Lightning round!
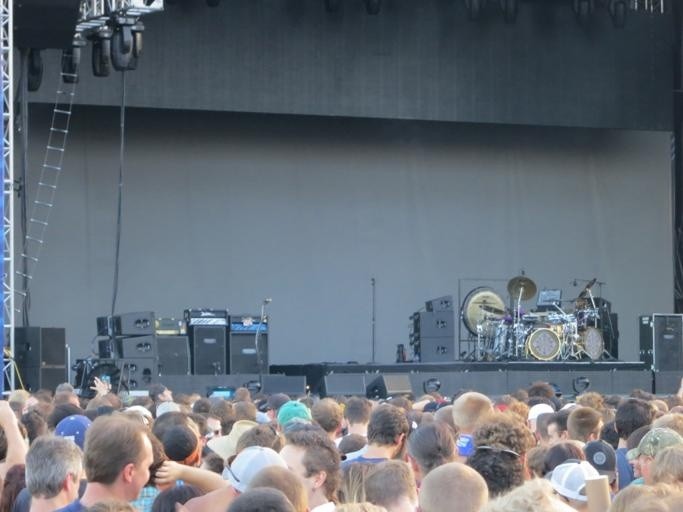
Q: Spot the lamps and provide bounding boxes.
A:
[25,0,143,95]
[362,0,628,31]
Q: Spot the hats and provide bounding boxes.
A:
[206,394,314,492]
[625,428,683,461]
[528,403,553,421]
[550,459,597,501]
[54,414,92,449]
[8,390,38,406]
[585,439,616,484]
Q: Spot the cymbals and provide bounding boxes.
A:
[479,305,504,314]
[507,277,536,300]
[577,278,596,298]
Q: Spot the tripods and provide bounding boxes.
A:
[464,286,532,363]
[551,286,619,361]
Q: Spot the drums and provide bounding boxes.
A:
[525,327,561,360]
[567,322,578,335]
[544,322,563,337]
[578,326,604,360]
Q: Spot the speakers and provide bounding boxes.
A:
[259,374,307,395]
[367,373,413,400]
[310,372,368,400]
[157,335,192,375]
[96,310,160,391]
[227,330,270,376]
[14,326,69,397]
[188,324,228,375]
[413,295,456,363]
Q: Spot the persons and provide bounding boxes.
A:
[1,375,683,510]
[562,297,586,313]
